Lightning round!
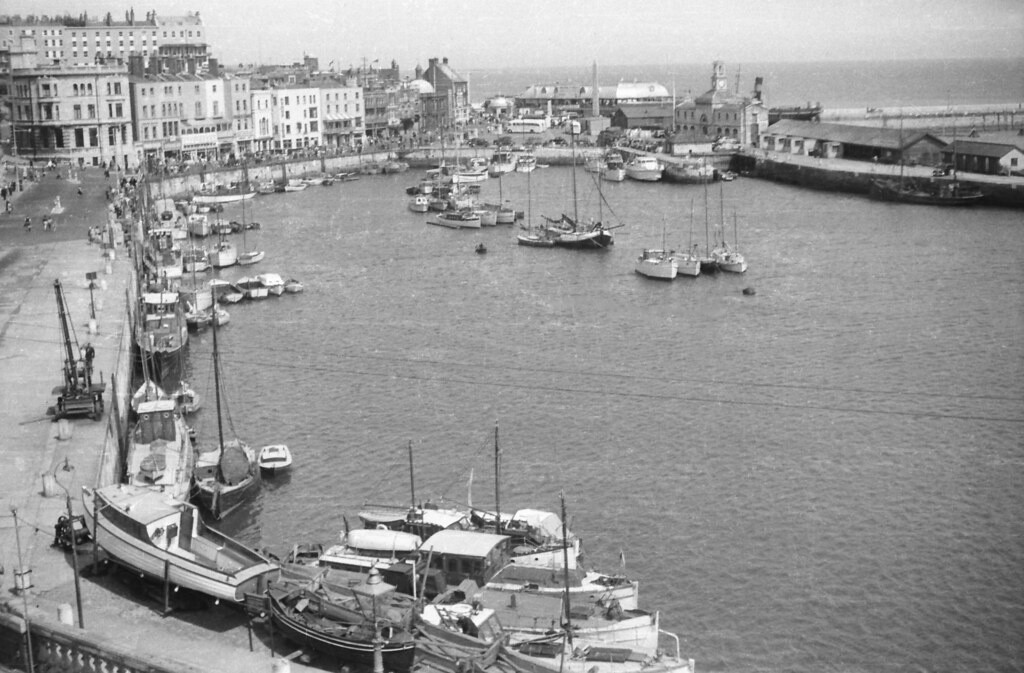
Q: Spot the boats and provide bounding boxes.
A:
[870,102,986,205]
[636,248,680,279]
[673,249,704,278]
[78,483,281,606]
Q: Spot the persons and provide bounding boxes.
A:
[527,116,698,171]
[0,151,141,243]
[84,342,95,376]
[856,149,924,175]
[455,611,479,639]
[146,101,515,181]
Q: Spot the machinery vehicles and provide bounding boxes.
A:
[50,277,107,417]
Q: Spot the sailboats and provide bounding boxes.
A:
[692,178,714,271]
[139,98,735,673]
[709,180,749,273]
